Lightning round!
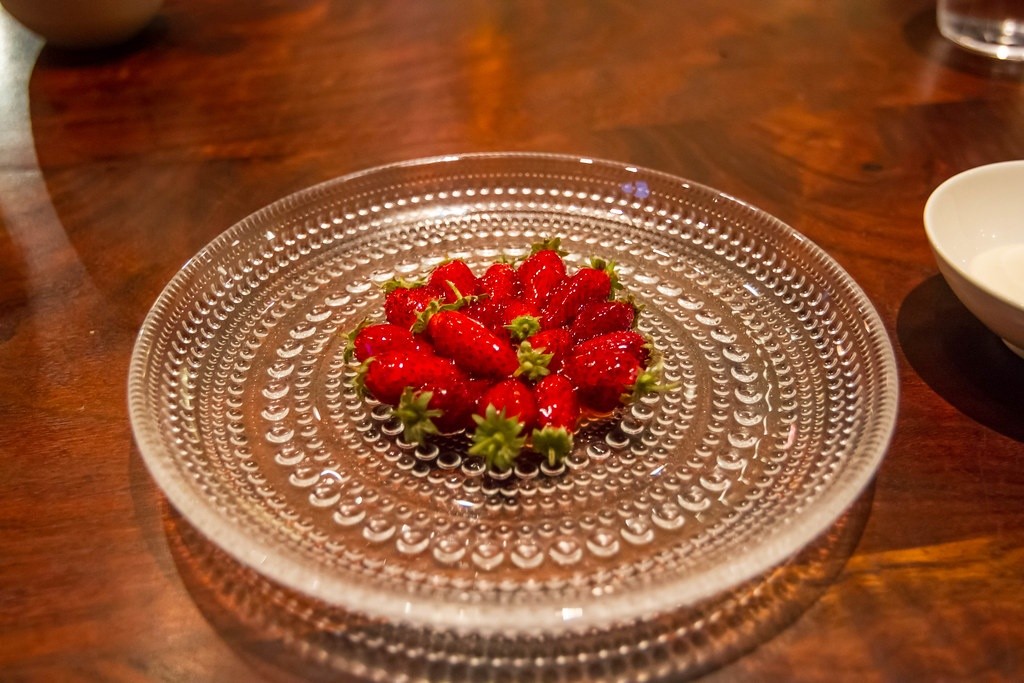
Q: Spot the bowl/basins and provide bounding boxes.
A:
[923,160,1024,359]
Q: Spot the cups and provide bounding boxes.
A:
[937,0,1024,61]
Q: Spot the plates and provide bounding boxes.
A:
[126,151,901,642]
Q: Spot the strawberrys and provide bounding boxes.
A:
[343,236,682,474]
